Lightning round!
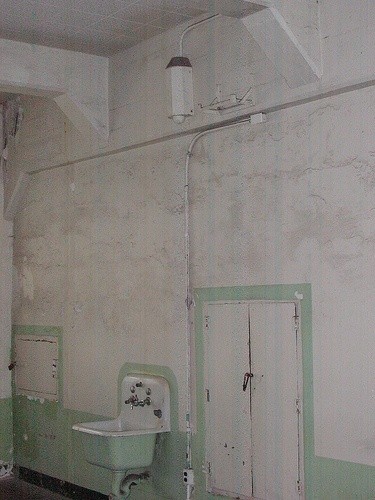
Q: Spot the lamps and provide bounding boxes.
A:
[165,57,194,124]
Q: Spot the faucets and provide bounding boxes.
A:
[129,399,145,410]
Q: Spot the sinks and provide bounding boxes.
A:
[71,418,163,472]
